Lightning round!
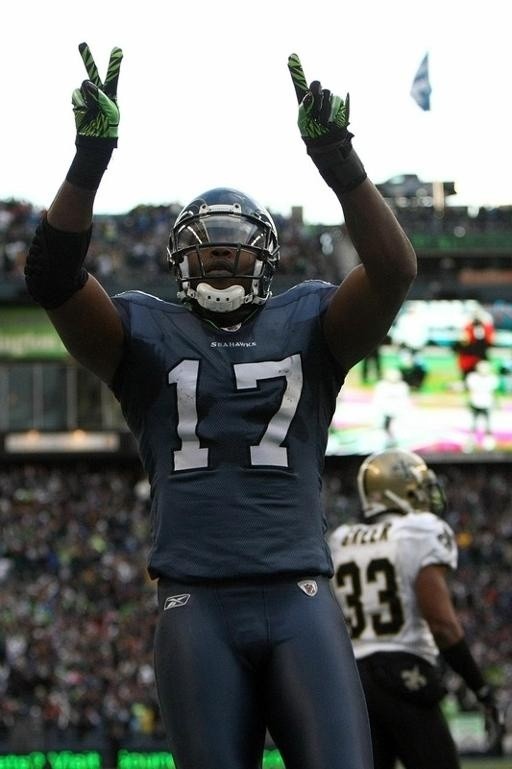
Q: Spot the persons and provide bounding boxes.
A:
[1,197,510,304]
[356,297,501,437]
[26,40,419,769]
[1,446,510,732]
[320,448,507,769]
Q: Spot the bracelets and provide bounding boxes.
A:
[65,146,115,192]
[308,146,367,194]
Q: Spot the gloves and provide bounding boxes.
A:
[289,53,366,193]
[65,42,123,190]
[475,687,503,752]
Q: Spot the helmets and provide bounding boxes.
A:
[165,188,281,333]
[359,450,448,520]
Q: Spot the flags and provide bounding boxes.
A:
[408,57,434,108]
